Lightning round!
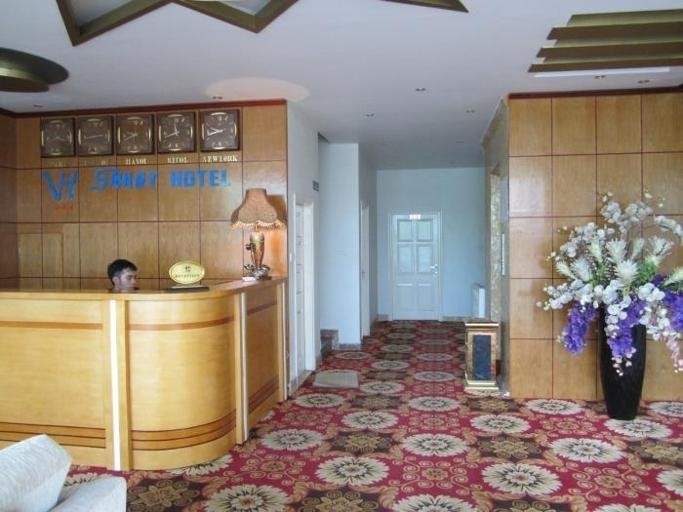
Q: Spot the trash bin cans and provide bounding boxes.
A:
[463,318,500,392]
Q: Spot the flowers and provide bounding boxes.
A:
[532,187,683,377]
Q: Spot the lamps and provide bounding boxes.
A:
[230,186,284,278]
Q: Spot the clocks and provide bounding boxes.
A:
[35,109,242,159]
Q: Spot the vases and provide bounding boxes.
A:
[600,295,646,420]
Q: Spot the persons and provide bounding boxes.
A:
[107,258,138,292]
